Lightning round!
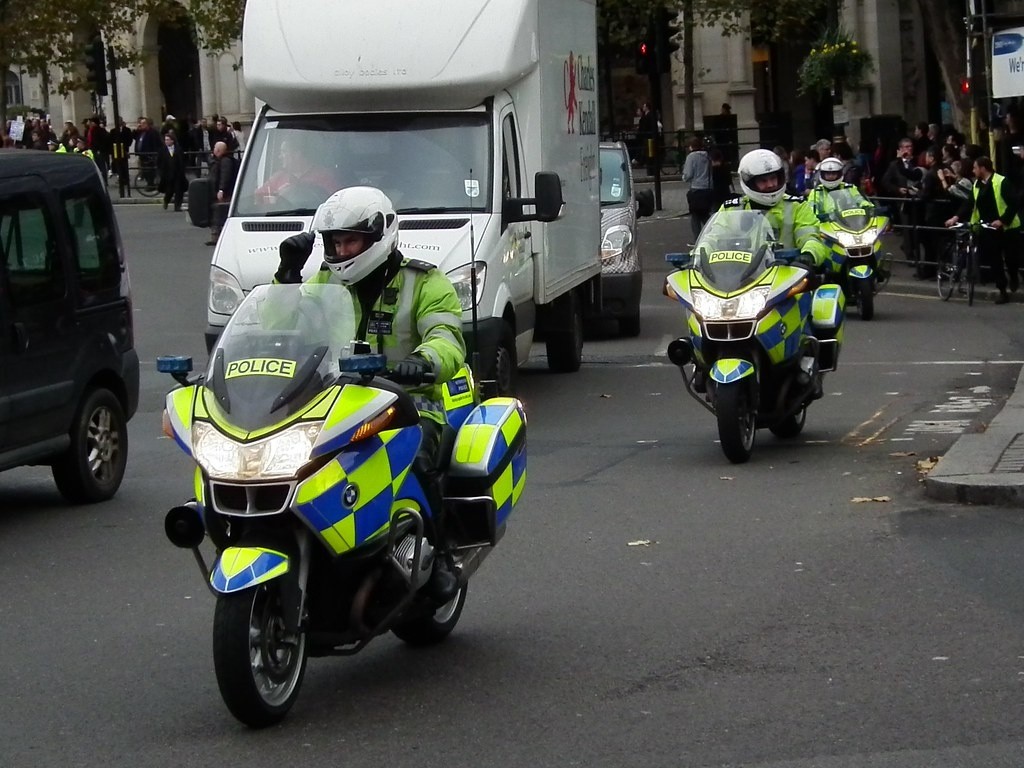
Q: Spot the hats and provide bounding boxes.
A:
[47,136,60,145]
[166,115,176,120]
[77,135,88,143]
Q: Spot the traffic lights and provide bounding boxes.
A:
[638,42,650,72]
[660,8,680,54]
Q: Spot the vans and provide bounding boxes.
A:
[0,150,140,505]
[599,140,654,335]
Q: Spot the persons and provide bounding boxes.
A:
[772,110,1024,304]
[720,103,731,115]
[0,108,246,211]
[255,137,342,197]
[631,102,658,168]
[688,149,827,270]
[261,186,467,596]
[204,141,239,245]
[682,137,730,249]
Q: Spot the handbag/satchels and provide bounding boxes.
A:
[686,188,712,213]
[231,130,239,150]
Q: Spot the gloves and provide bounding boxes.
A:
[798,252,814,267]
[392,351,432,387]
[274,232,315,284]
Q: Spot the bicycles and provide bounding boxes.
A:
[660,135,688,175]
[938,221,996,306]
[135,170,161,197]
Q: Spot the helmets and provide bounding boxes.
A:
[317,186,399,287]
[738,149,786,207]
[819,157,843,190]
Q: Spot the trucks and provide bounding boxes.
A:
[186,0,602,395]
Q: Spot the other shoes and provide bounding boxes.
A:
[687,240,695,247]
[205,241,216,245]
[1010,275,1018,292]
[800,361,823,400]
[996,293,1009,304]
[175,207,182,211]
[164,200,167,208]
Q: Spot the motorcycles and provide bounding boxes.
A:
[155,348,527,730]
[814,189,890,321]
[661,211,846,463]
